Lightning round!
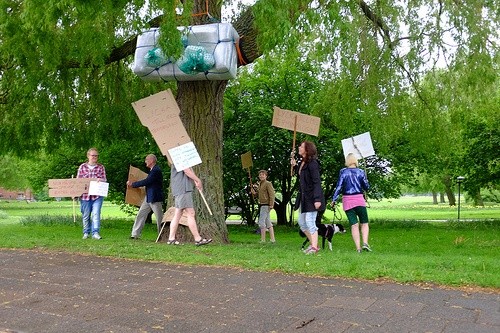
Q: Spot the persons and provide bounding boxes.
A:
[250,170,276,246]
[125,154,166,243]
[290,141,322,256]
[328,153,373,254]
[76,146,108,240]
[165,163,213,247]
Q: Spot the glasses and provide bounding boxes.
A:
[90,154,99,157]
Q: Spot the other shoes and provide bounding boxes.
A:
[258,239,266,243]
[267,240,275,245]
[82,233,89,239]
[357,249,361,252]
[362,243,372,252]
[93,232,102,240]
[306,247,319,256]
[304,245,312,254]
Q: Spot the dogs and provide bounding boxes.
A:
[299,223,347,251]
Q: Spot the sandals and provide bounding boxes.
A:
[167,239,184,246]
[194,238,213,246]
